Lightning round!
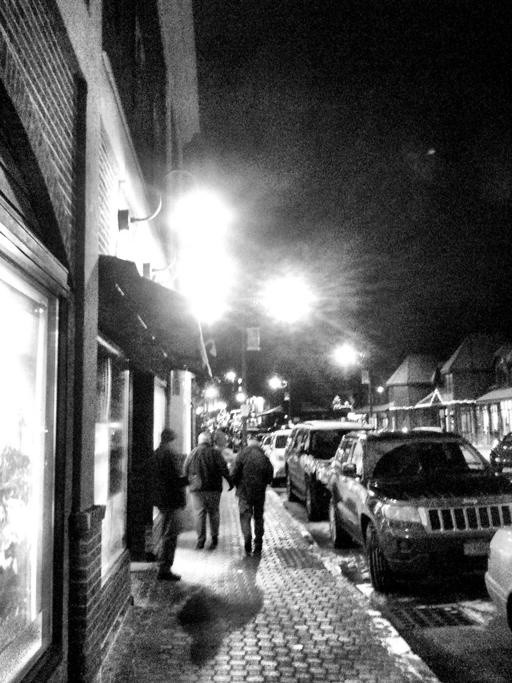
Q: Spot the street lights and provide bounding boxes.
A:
[179,253,317,426]
[332,343,372,414]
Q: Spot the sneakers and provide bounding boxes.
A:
[198,536,218,549]
[245,534,262,553]
[157,571,181,580]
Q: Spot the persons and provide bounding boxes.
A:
[231,438,274,555]
[198,423,271,474]
[138,429,200,581]
[183,430,234,550]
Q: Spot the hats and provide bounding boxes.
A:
[161,428,178,444]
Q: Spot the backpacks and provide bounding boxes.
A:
[130,450,173,505]
[242,444,270,498]
